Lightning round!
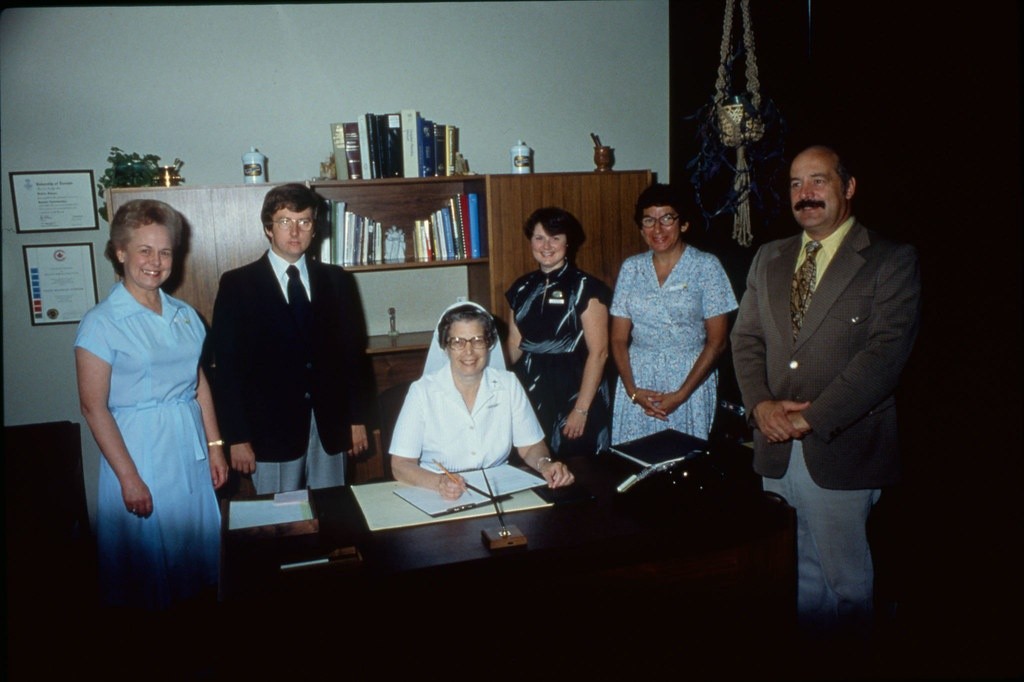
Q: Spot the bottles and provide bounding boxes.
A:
[510,140,532,174]
[242,146,267,184]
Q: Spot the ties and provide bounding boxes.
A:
[286,265,310,324]
[791,241,823,342]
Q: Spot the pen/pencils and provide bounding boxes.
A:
[432,457,472,496]
[280,559,330,569]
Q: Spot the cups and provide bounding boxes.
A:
[593,146,612,172]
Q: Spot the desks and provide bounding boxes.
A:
[216,454,801,682]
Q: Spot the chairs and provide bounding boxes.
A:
[373,381,412,481]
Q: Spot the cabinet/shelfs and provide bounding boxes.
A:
[108,168,654,482]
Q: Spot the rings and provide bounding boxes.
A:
[132,509,136,512]
[359,447,363,448]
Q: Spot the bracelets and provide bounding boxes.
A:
[575,408,587,415]
[632,389,639,400]
[537,456,551,469]
[208,440,223,446]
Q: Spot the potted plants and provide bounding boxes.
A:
[97,145,160,224]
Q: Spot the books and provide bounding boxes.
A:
[414,193,480,258]
[331,110,456,181]
[319,200,382,264]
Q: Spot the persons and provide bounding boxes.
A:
[388,301,575,501]
[73,198,229,609]
[206,182,376,495]
[730,145,922,632]
[505,206,612,456]
[609,183,740,445]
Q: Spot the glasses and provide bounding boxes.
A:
[449,335,488,350]
[271,218,313,231]
[640,214,680,227]
